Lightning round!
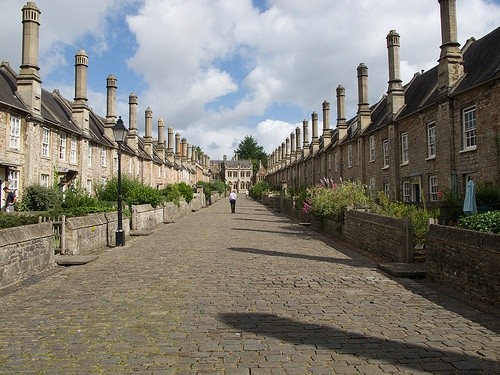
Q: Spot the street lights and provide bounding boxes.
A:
[207,168,212,206]
[111,116,126,246]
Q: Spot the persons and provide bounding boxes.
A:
[244,189,249,198]
[2,186,17,211]
[229,190,237,214]
